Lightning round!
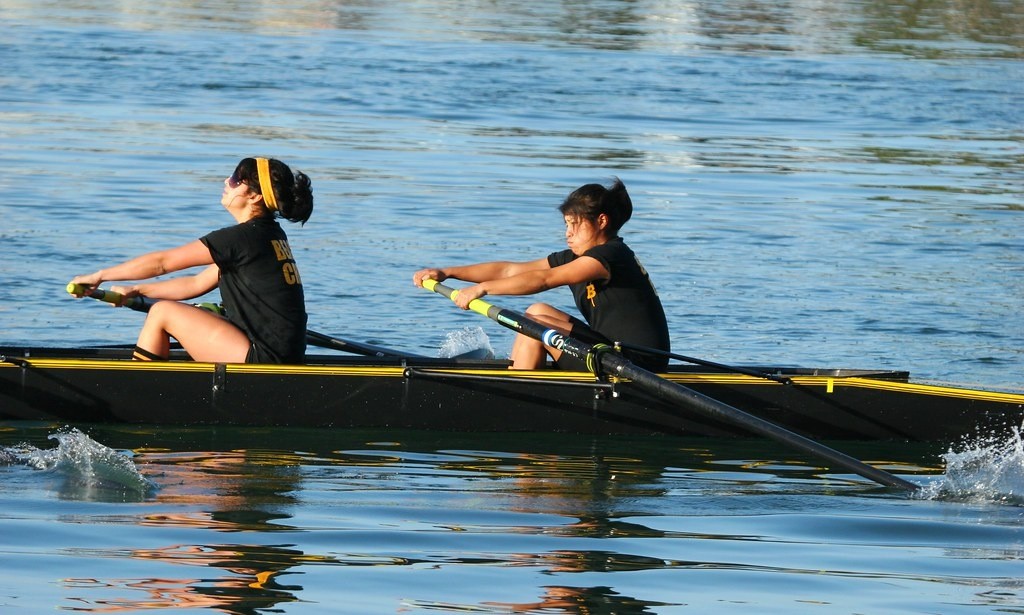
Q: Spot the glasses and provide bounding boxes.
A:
[229,174,261,196]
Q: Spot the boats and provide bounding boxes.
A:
[0,331,1024,463]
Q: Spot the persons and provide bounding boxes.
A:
[412,176,671,375]
[66,155,313,366]
